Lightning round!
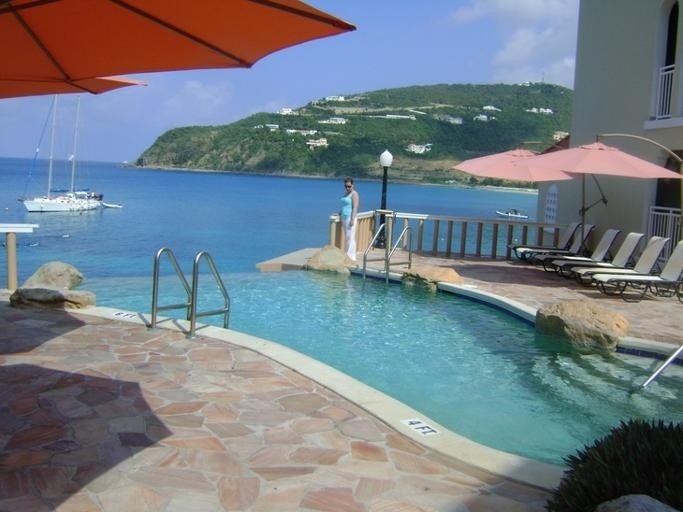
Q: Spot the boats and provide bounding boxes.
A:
[494,207,528,220]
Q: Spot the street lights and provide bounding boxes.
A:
[373,148,393,250]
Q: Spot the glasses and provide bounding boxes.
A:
[344,185,352,189]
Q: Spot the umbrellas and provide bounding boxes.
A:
[459,138,608,255]
[0,74,140,106]
[511,133,683,242]
[0,0,360,82]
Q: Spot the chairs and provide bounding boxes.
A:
[505,221,683,303]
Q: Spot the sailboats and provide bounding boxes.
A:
[18,92,122,213]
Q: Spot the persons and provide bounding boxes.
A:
[338,176,360,262]
